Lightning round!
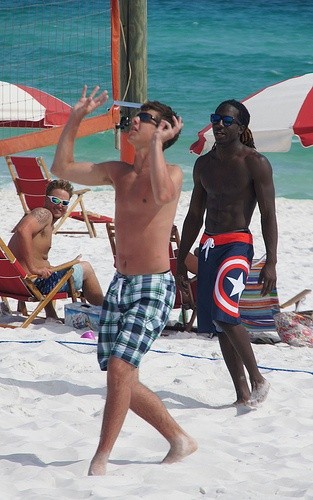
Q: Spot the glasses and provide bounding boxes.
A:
[48,196,70,206]
[211,114,243,127]
[137,113,158,122]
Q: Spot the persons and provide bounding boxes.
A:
[8,180,104,307]
[51,84,197,476]
[176,100,278,408]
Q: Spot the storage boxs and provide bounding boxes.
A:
[64,302,103,332]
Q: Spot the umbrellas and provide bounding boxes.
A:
[189,73,313,157]
[0,81,74,128]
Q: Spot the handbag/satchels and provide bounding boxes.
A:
[273,310,313,348]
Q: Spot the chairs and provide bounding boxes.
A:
[0,154,313,347]
